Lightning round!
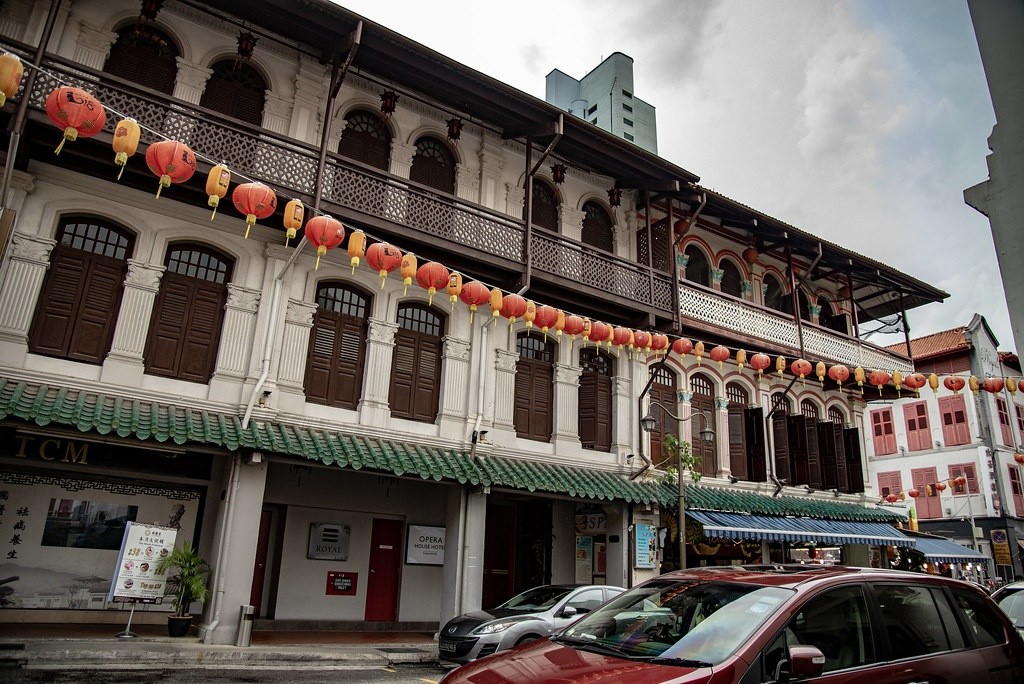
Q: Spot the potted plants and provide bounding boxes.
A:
[154,539,212,636]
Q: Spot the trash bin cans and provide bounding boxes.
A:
[234,605,255,646]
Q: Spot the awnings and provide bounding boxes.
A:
[900,536,990,564]
[685,509,917,547]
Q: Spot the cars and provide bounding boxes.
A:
[438,564,1024,684]
[436,582,682,667]
[968,581,1024,640]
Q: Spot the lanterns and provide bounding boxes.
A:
[1014,451,1024,465]
[673,218,690,234]
[606,187,622,208]
[839,285,853,301]
[112,117,141,180]
[138,0,164,23]
[743,248,757,264]
[786,265,802,279]
[206,162,980,412]
[550,162,568,185]
[378,87,401,117]
[1006,377,1018,396]
[885,475,968,507]
[1018,378,1024,394]
[0,51,25,112]
[46,86,106,155]
[983,377,1004,397]
[445,116,465,141]
[146,140,196,199]
[236,31,259,60]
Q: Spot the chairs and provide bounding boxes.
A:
[800,596,856,669]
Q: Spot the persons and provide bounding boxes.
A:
[902,560,990,587]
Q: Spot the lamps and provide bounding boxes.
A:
[899,445,904,452]
[446,101,465,146]
[236,19,260,63]
[805,486,816,493]
[260,391,272,405]
[906,556,982,571]
[833,489,842,499]
[480,429,489,441]
[727,475,739,484]
[378,68,400,115]
[936,441,942,447]
[607,178,623,208]
[628,454,635,464]
[946,508,951,514]
[550,145,568,183]
[779,479,787,487]
[134,0,165,32]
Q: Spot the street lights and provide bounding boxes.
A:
[640,401,715,571]
